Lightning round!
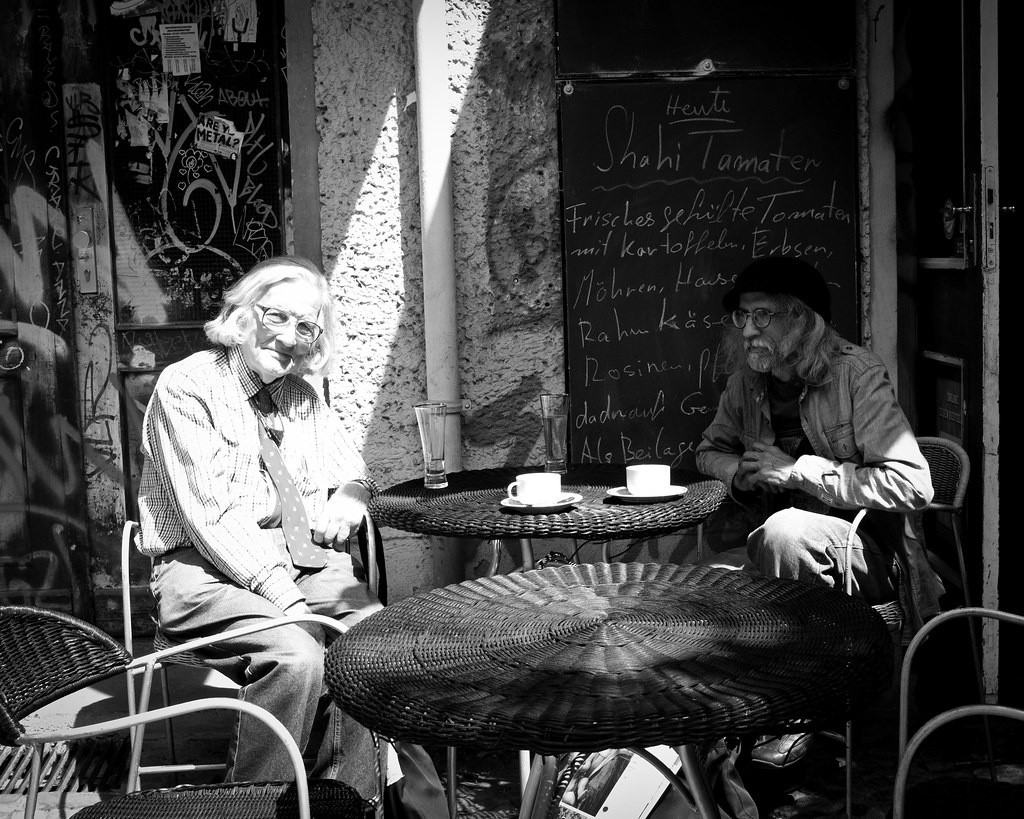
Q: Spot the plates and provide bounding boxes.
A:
[500,492,584,514]
[606,485,688,502]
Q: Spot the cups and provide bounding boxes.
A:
[507,472,561,505]
[539,393,572,474]
[414,403,449,489]
[625,464,671,496]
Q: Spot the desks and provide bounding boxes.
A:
[370,456,729,576]
[327,560,895,819]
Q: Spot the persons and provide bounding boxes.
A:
[691,255,946,819]
[134,257,385,819]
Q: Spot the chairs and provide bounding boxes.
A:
[119,499,381,793]
[0,603,386,819]
[896,605,1024,819]
[826,436,999,819]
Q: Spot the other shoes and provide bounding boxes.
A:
[751,718,817,768]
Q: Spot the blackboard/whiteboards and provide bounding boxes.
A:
[553,73,868,468]
[95,0,292,331]
[2,1,123,626]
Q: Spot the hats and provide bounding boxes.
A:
[725,256,831,327]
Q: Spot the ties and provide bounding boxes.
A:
[256,388,328,567]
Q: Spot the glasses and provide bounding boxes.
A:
[253,302,323,344]
[732,307,792,330]
